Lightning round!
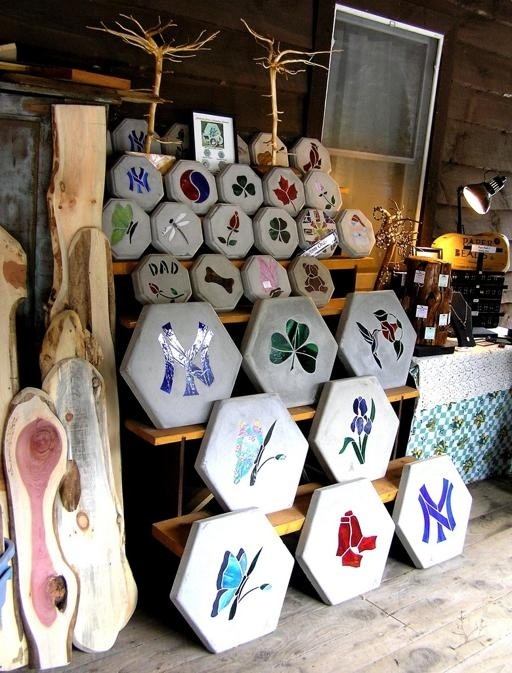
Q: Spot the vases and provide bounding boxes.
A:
[125,150,175,177]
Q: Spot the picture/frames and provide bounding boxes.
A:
[188,109,241,173]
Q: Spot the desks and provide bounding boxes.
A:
[411,325,511,485]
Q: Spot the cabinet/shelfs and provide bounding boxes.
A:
[105,256,421,563]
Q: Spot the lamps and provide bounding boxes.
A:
[457,172,507,236]
